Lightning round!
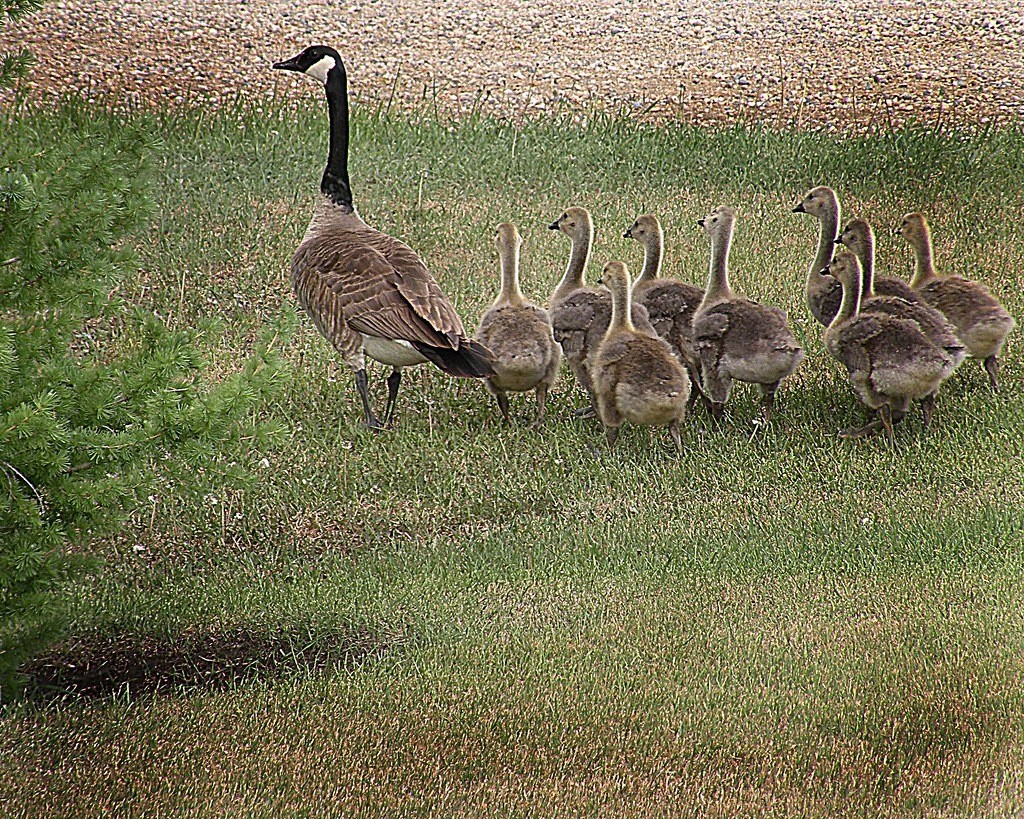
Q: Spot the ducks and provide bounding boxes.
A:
[820,250,953,450]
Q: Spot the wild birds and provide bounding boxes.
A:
[793,185,949,328]
[548,206,661,420]
[479,223,561,429]
[592,261,692,456]
[892,210,1015,393]
[690,204,802,433]
[272,45,496,434]
[621,215,710,409]
[831,215,967,442]
